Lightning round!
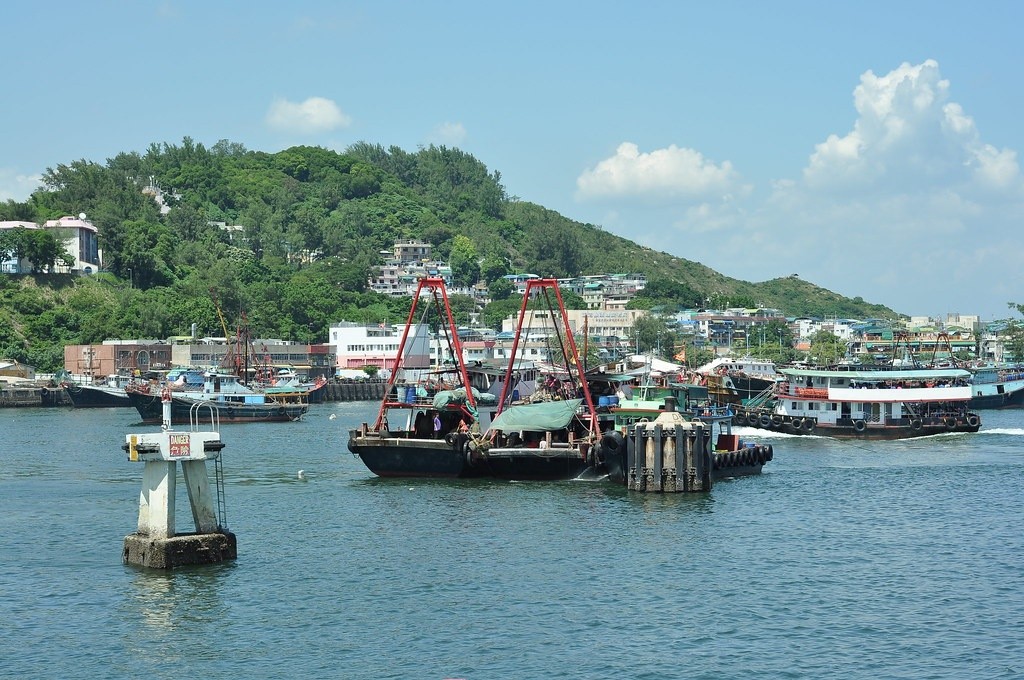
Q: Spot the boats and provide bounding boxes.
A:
[58,306,328,405]
[345,275,1023,480]
[122,375,311,424]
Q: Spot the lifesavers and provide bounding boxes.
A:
[792,418,815,431]
[227,407,233,413]
[854,420,867,433]
[735,412,782,427]
[587,447,594,467]
[347,440,357,454]
[601,431,625,455]
[463,441,469,457]
[911,416,980,431]
[716,444,774,467]
[503,436,512,448]
[467,451,475,468]
[277,406,286,415]
[445,433,456,446]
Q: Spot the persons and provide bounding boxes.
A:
[457,420,468,432]
[527,435,562,448]
[912,405,967,417]
[978,390,982,396]
[998,369,1024,381]
[849,382,868,389]
[433,413,442,439]
[877,378,902,389]
[473,422,481,433]
[904,379,968,388]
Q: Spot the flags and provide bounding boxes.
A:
[674,350,685,363]
